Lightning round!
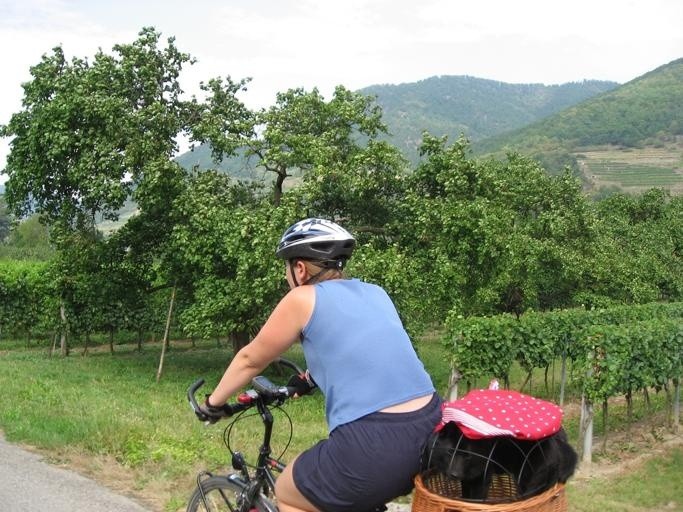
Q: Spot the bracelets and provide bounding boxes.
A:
[204,393,226,410]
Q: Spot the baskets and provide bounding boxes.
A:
[411,471,568,512]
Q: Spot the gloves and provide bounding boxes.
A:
[194,393,228,424]
[288,368,317,398]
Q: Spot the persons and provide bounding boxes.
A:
[194,216,447,512]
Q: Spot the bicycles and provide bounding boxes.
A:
[186,358,388,512]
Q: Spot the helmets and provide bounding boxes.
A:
[275,218,356,260]
